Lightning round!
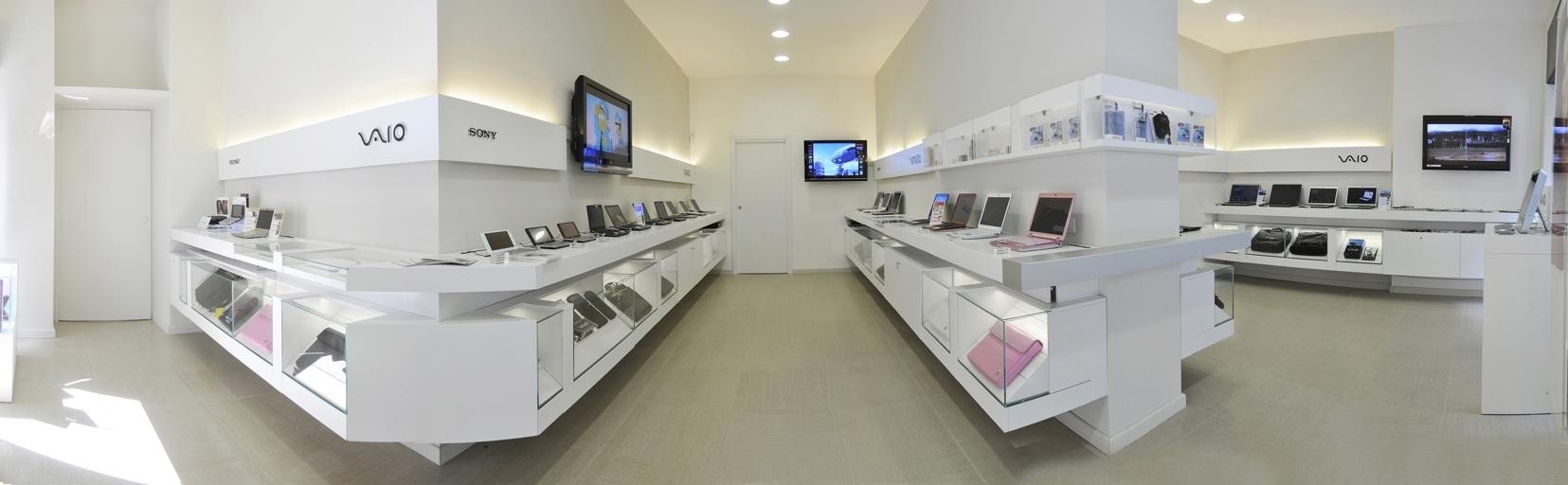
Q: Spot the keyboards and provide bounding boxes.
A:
[1493,222,1514,233]
[557,290,616,337]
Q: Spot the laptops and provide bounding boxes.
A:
[219,204,274,238]
[907,193,1076,251]
[859,191,902,215]
[1180,224,1202,231]
[481,199,716,256]
[1221,184,1379,209]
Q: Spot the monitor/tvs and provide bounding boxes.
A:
[1515,166,1548,234]
[804,140,868,182]
[575,75,636,175]
[1420,113,1512,173]
[1554,118,1568,173]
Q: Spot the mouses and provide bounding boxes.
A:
[1498,229,1515,235]
